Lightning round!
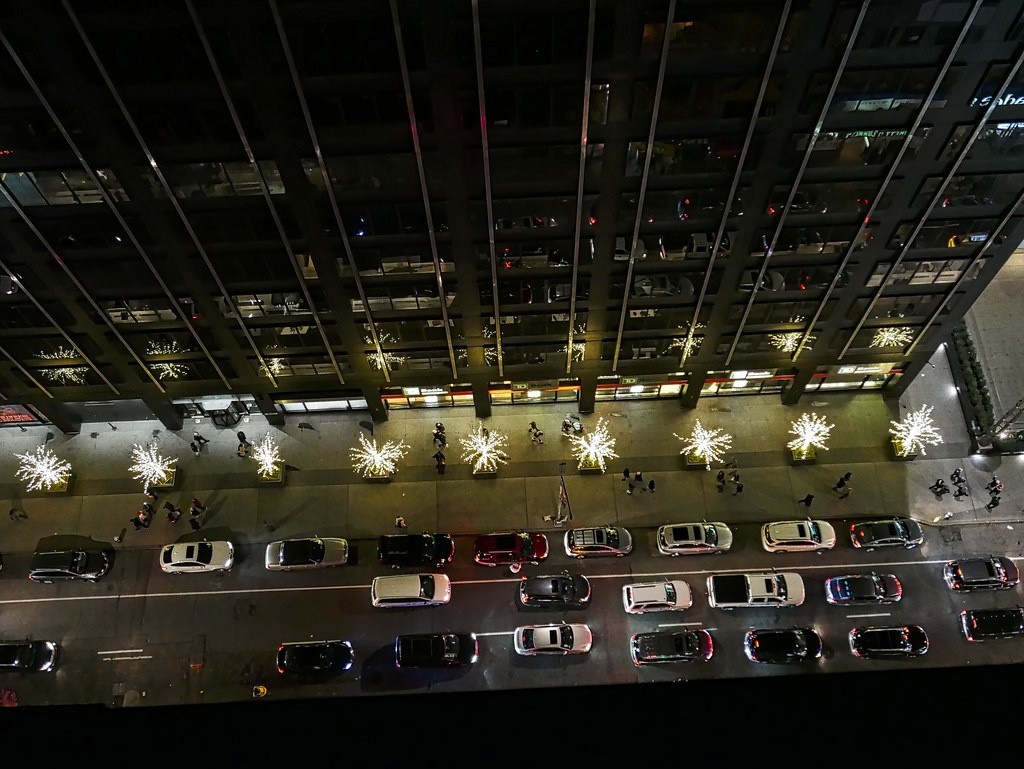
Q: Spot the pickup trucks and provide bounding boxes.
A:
[960,603,1024,642]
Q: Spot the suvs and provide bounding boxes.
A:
[623,575,693,615]
[375,531,455,570]
[372,572,452,608]
[28,549,110,584]
[472,531,549,565]
[759,514,837,556]
[518,568,592,606]
[706,566,807,612]
[942,555,1020,595]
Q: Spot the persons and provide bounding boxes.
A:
[6,407,1011,544]
[252,685,274,698]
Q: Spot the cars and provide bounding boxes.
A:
[392,628,481,670]
[263,533,349,574]
[158,536,234,575]
[0,634,59,673]
[826,570,903,606]
[849,511,925,553]
[741,625,824,665]
[656,518,733,557]
[514,618,592,658]
[274,637,354,679]
[561,524,633,560]
[629,623,714,668]
[847,622,930,661]
